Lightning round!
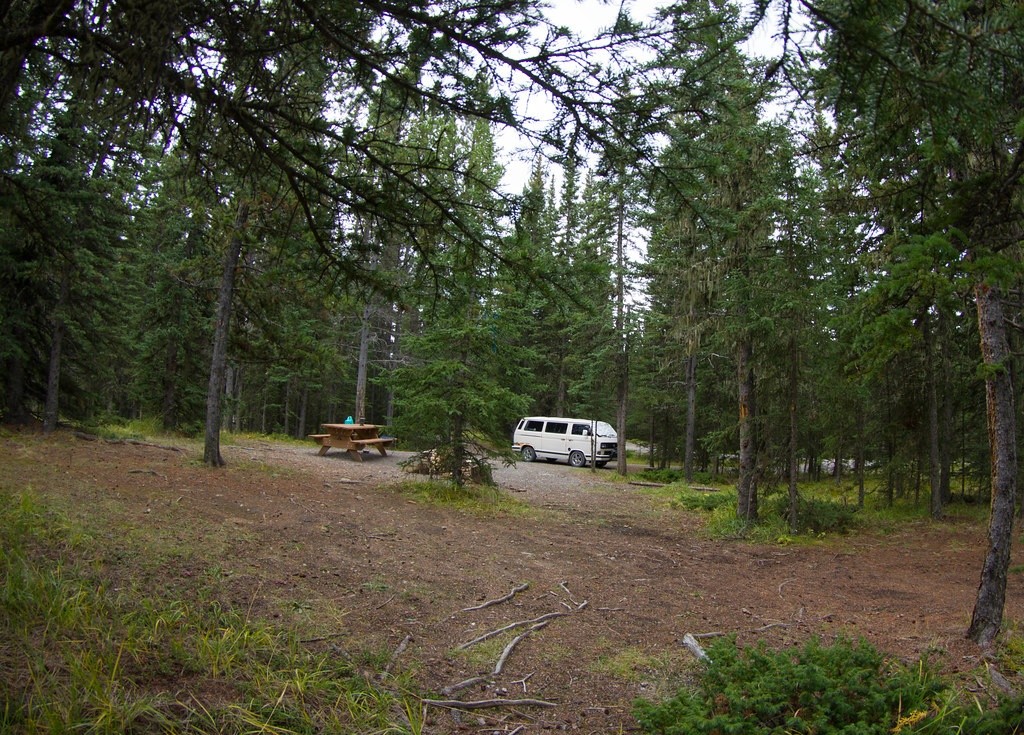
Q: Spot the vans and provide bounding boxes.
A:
[512,416,618,466]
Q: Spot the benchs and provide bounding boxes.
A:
[308,434,360,455]
[349,438,398,462]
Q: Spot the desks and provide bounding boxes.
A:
[321,423,386,461]
